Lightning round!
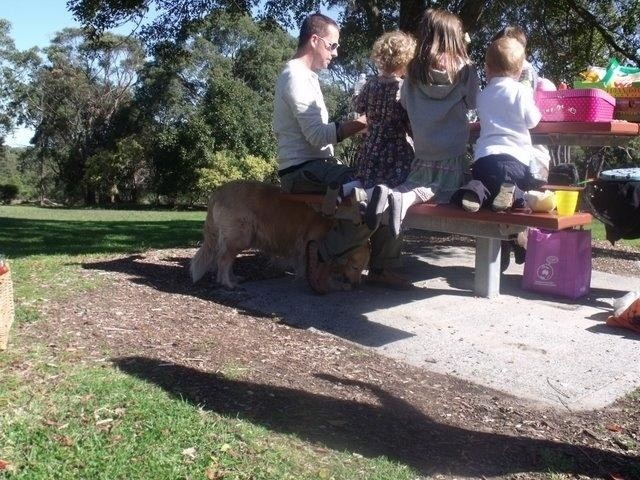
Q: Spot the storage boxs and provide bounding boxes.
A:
[572,80,640,122]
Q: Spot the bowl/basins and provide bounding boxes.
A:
[523,190,560,212]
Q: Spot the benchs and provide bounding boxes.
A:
[278,190,593,301]
[539,182,594,211]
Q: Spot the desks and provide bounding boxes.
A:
[466,117,640,188]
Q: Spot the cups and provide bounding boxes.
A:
[555,189,579,216]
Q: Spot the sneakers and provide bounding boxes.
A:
[491,181,516,213]
[360,266,413,291]
[321,180,343,220]
[501,237,522,271]
[350,184,403,239]
[460,189,481,214]
[306,240,332,296]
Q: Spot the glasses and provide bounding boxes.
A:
[317,35,341,51]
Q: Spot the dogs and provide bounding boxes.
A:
[188,179,372,295]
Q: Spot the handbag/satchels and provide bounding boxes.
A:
[521,225,592,299]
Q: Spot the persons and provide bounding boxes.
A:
[321,30,418,225]
[496,25,537,274]
[364,8,482,241]
[451,37,542,213]
[272,14,367,196]
